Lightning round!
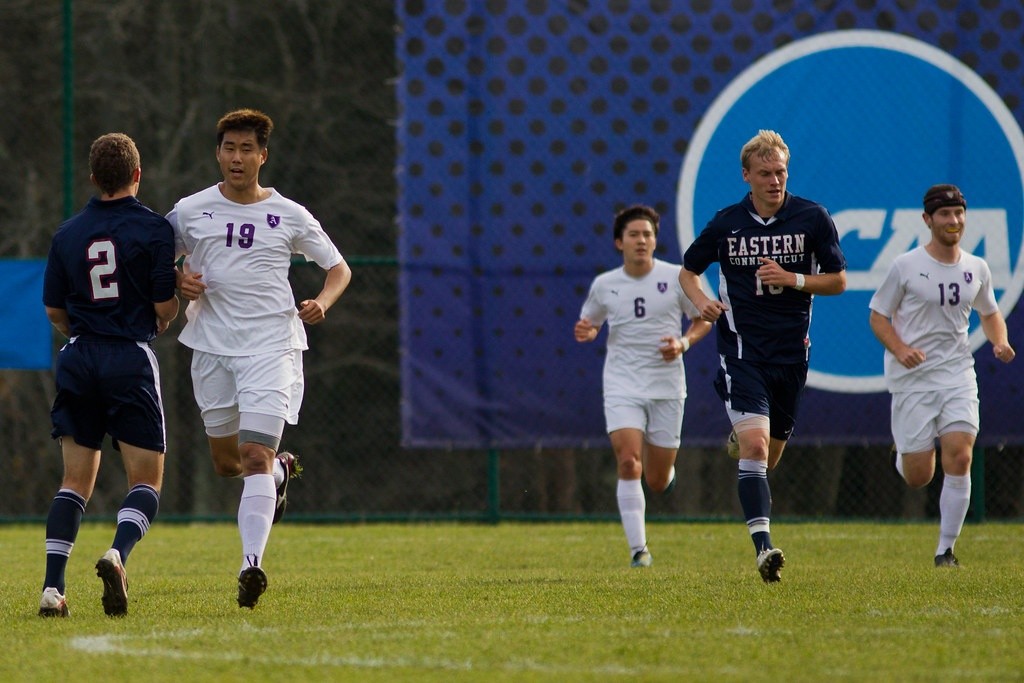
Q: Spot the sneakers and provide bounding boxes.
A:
[630,541,652,567]
[727,430,741,461]
[757,548,785,583]
[662,477,677,496]
[237,553,267,608]
[95,548,128,619]
[38,587,70,617]
[272,452,297,523]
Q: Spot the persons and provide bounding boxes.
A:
[677,129,848,582]
[165,109,353,608]
[38,131,181,617]
[571,204,712,568]
[868,184,1016,564]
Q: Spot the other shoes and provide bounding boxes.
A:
[934,549,958,567]
[890,443,904,482]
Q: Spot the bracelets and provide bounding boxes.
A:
[795,273,805,290]
[680,336,689,352]
